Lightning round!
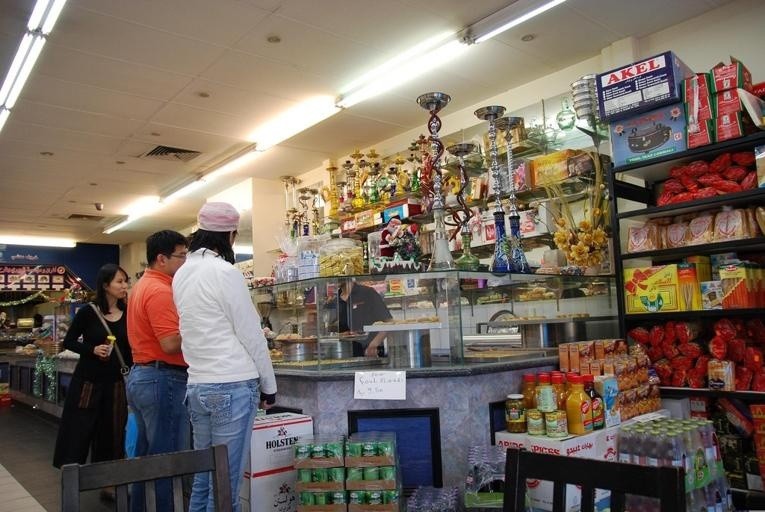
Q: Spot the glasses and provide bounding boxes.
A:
[170,253,188,260]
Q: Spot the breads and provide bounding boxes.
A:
[626,205,764,253]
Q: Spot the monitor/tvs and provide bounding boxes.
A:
[76,242,120,275]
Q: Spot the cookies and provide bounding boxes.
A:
[319,246,364,276]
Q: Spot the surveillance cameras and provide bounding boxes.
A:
[95,203,104,211]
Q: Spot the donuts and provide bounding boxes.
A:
[519,287,555,300]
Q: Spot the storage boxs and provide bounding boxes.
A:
[684,56,765,148]
[495,409,672,512]
[237,413,315,511]
[592,50,699,124]
[606,103,689,167]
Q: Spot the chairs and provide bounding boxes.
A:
[502,447,686,511]
[62,443,233,512]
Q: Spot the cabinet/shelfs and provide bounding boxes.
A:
[286,126,611,319]
[599,130,765,512]
[249,271,620,378]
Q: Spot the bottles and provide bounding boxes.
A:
[616,419,735,512]
[555,96,575,129]
[319,237,364,277]
[522,369,605,435]
[461,445,532,512]
[99,336,116,362]
[404,485,461,512]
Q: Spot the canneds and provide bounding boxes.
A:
[505,385,568,439]
[296,431,397,506]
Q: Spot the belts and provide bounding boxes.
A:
[136,363,152,367]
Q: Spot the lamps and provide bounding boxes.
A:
[103,142,260,239]
[462,0,566,47]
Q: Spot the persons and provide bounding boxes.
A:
[53,263,131,502]
[324,278,395,358]
[124,229,189,512]
[172,202,278,512]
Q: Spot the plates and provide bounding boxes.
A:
[486,310,514,335]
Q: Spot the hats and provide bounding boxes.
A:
[197,201,241,236]
